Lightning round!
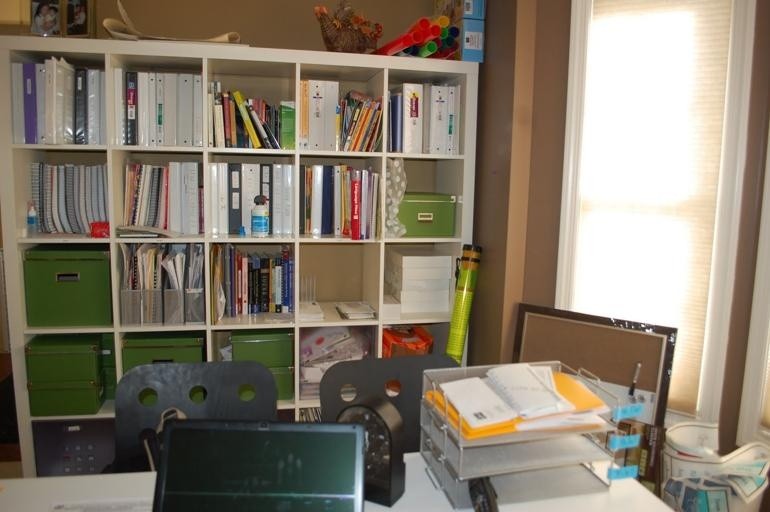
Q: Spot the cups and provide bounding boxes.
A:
[90,219,109,240]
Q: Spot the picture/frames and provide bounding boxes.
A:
[512,303,678,427]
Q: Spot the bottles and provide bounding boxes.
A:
[26,201,39,237]
[251,194,268,238]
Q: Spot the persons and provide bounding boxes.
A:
[32,2,87,39]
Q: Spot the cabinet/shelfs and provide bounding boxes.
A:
[0,36,479,478]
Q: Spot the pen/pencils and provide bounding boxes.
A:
[629,362,643,396]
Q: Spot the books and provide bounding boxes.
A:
[300,164,381,241]
[120,243,204,324]
[32,163,109,233]
[299,408,321,422]
[336,304,376,320]
[299,303,323,322]
[427,363,611,439]
[388,83,459,154]
[114,67,203,146]
[210,245,293,325]
[11,59,107,144]
[118,160,202,239]
[206,80,295,149]
[210,162,293,235]
[300,80,383,151]
[300,383,356,400]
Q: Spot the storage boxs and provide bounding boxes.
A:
[122,334,205,406]
[23,245,112,327]
[397,191,457,237]
[230,330,294,402]
[24,336,116,415]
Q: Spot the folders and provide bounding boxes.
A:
[11,57,106,145]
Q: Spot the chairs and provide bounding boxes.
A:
[115,360,277,473]
[320,354,461,454]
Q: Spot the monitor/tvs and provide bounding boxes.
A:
[152,417,366,512]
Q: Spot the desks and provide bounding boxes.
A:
[1,453,677,512]
